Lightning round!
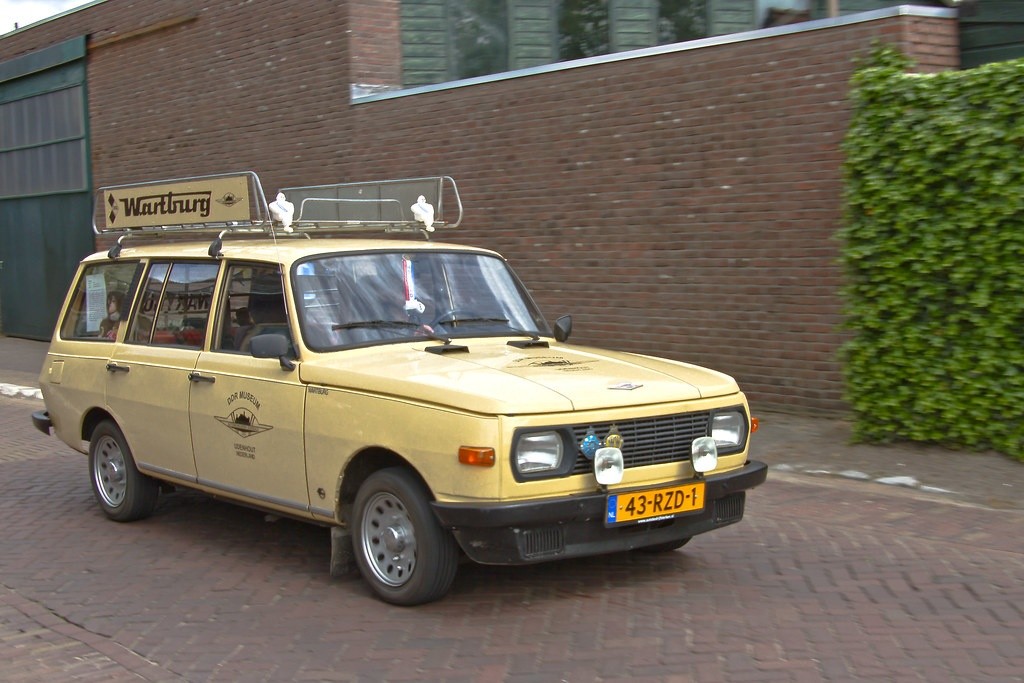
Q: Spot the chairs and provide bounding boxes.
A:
[241,307,293,353]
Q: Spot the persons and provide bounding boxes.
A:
[356,268,449,342]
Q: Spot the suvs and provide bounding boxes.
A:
[29,170,769,607]
